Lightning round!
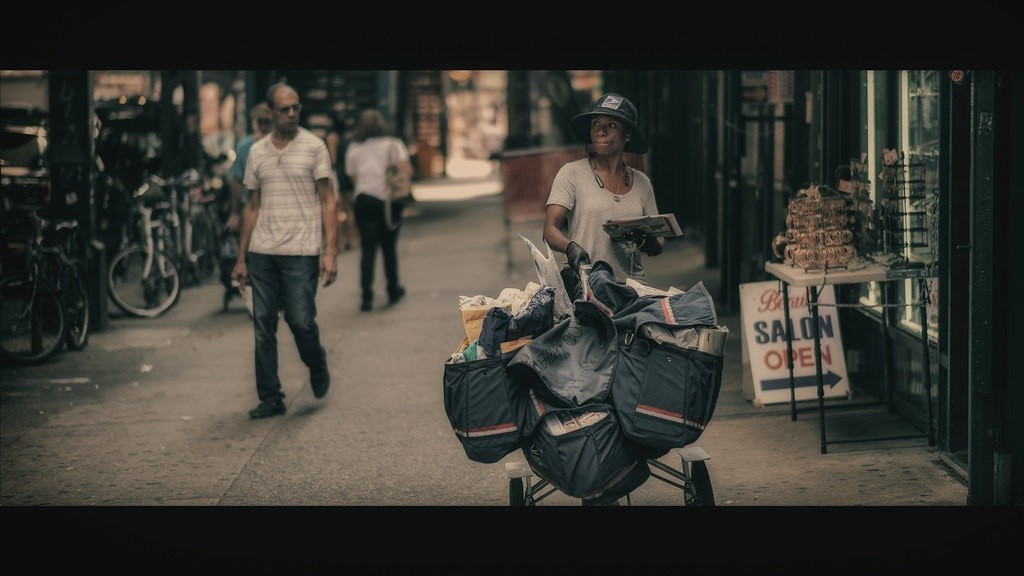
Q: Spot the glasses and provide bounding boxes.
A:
[274,103,303,114]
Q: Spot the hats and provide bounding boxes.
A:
[250,102,271,119]
[570,93,650,155]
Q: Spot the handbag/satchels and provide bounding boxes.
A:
[385,134,412,198]
[560,260,640,324]
[442,287,558,464]
[611,279,725,451]
[505,300,646,498]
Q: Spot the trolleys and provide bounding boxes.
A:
[504,266,717,508]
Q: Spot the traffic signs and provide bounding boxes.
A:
[739,280,852,405]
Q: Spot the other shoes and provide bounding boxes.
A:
[309,346,330,399]
[249,401,286,418]
[389,287,404,305]
[362,289,373,311]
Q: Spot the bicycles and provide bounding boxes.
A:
[96,149,240,319]
[0,211,91,367]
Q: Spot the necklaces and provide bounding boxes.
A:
[591,154,631,202]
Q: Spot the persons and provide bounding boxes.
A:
[541,95,668,285]
[230,84,339,418]
[346,109,415,309]
[222,103,272,226]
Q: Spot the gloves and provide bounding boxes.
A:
[566,241,591,275]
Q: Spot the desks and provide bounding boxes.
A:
[766,262,937,454]
